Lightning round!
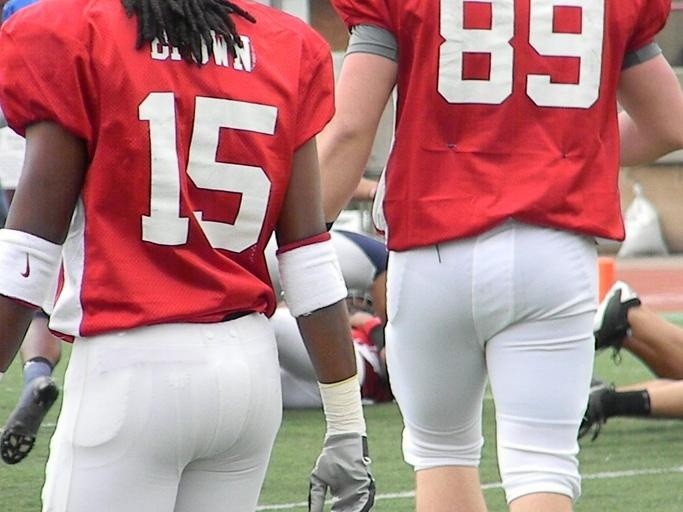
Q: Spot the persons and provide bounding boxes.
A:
[265,178,391,408]
[0,0,377,512]
[316,0,683,512]
[576,281,683,440]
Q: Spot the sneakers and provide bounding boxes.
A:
[574,378,614,445]
[589,278,643,367]
[0,374,60,466]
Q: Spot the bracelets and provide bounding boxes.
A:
[317,374,366,434]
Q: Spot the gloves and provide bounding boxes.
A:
[304,427,376,512]
